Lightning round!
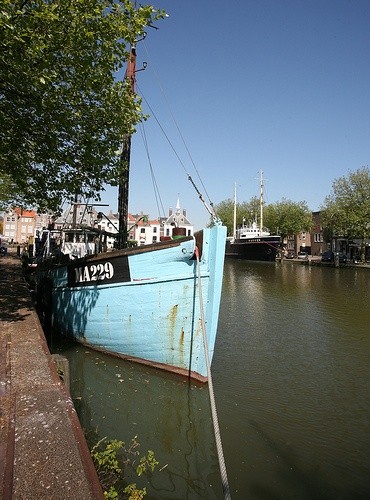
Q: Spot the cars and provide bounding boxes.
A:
[322,251,334,262]
[338,255,347,263]
[298,252,308,259]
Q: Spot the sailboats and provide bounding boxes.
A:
[231,170,282,262]
[29,36,227,384]
